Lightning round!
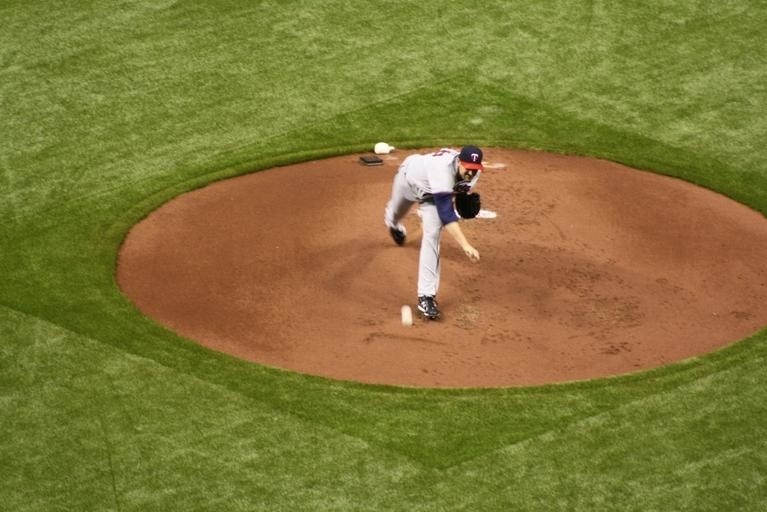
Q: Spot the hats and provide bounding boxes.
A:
[460,147,485,172]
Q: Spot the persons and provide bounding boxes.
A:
[384,145,483,321]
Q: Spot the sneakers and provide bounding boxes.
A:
[388,223,407,245]
[416,296,439,318]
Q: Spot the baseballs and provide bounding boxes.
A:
[401,305,413,325]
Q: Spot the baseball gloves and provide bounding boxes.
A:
[455,192,481,219]
